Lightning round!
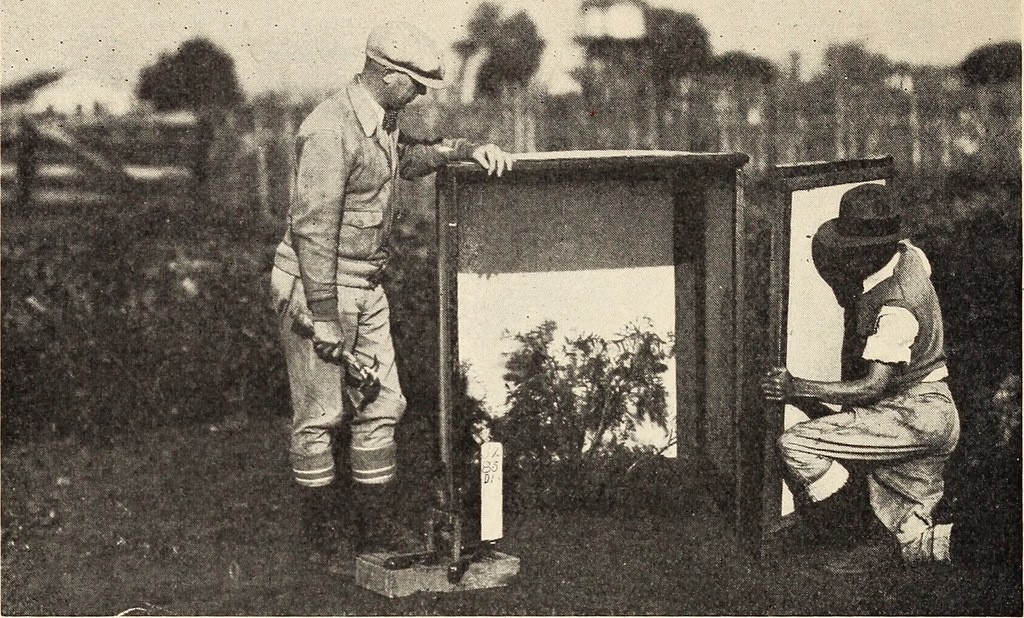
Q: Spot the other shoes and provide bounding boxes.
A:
[349,508,425,555]
[296,517,362,566]
[947,531,1011,612]
[817,532,906,577]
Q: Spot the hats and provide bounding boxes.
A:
[816,182,911,249]
[364,29,451,90]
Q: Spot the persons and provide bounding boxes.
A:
[758,182,997,570]
[269,18,515,564]
